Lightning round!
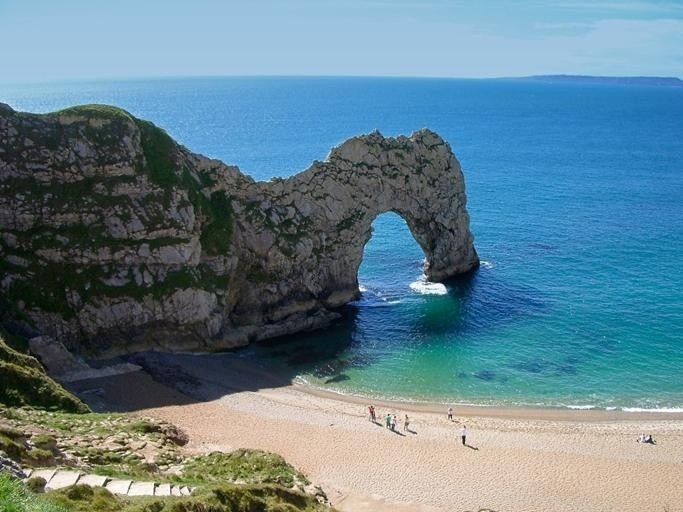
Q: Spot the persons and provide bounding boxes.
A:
[368,406,409,431]
[459,425,467,445]
[637,433,652,443]
[447,407,453,420]
